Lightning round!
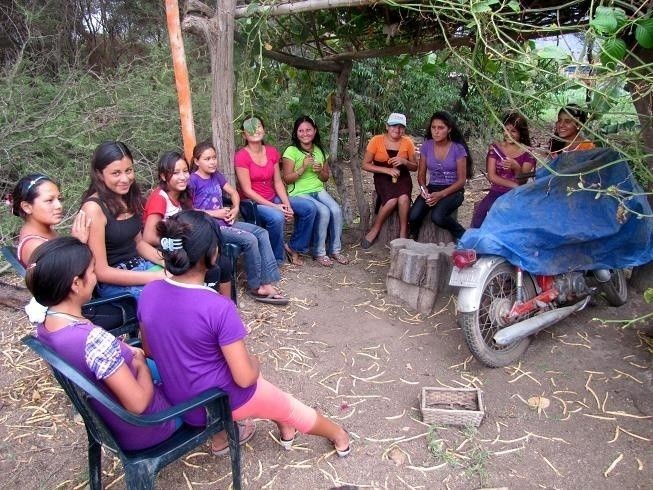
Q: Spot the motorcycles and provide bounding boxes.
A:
[444,169,629,368]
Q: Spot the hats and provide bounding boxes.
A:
[386,113,405,126]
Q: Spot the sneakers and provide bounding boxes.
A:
[360,238,371,248]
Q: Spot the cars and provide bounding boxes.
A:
[559,65,591,85]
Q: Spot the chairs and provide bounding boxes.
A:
[21,331,245,490]
[221,196,258,227]
[219,243,242,274]
[0,243,140,339]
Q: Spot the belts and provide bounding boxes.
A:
[125,257,140,270]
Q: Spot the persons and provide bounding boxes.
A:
[187,142,290,304]
[361,112,418,249]
[79,140,174,298]
[137,209,352,459]
[235,115,318,264]
[12,172,137,329]
[25,235,256,454]
[546,104,596,160]
[471,113,534,229]
[410,112,468,239]
[282,115,349,268]
[142,150,232,296]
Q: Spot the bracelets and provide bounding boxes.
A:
[296,171,301,176]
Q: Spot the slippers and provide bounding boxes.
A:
[211,420,255,455]
[286,252,300,263]
[329,255,348,264]
[248,290,288,304]
[314,256,332,266]
